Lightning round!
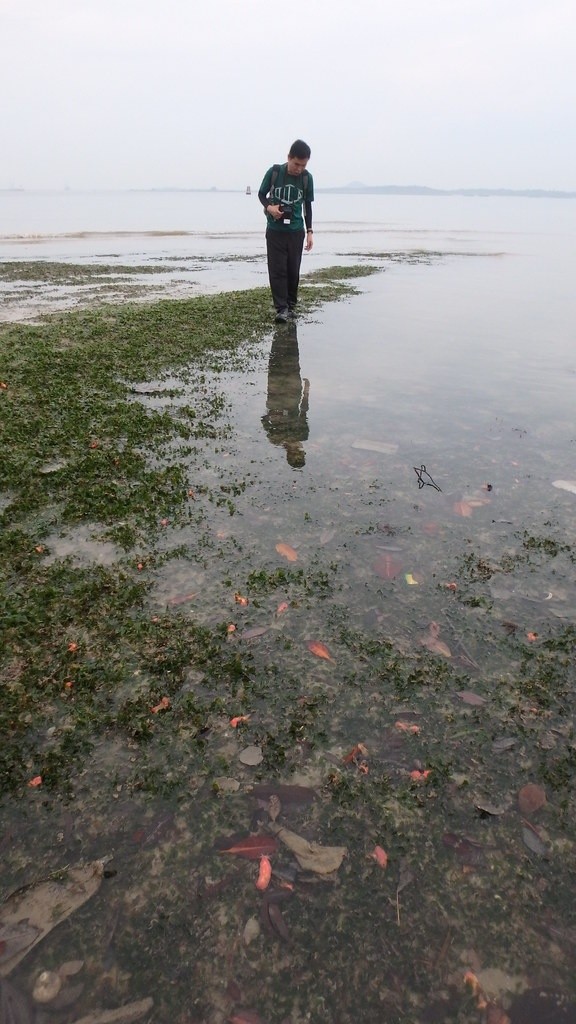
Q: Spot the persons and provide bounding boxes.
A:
[259,321,311,469]
[257,139,315,324]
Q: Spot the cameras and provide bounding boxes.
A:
[280,206,295,220]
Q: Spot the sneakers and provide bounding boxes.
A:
[275,308,288,321]
[287,310,300,318]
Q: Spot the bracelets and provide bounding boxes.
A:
[307,230,314,233]
